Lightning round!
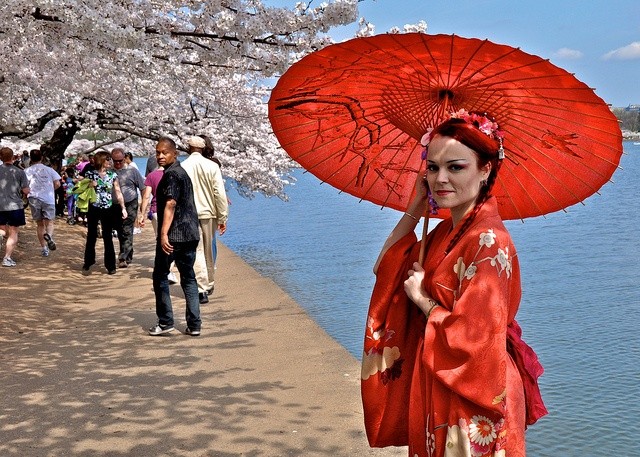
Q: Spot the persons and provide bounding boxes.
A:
[80,151,129,275]
[124,151,141,172]
[146,137,203,336]
[360,108,550,457]
[110,147,147,268]
[211,157,232,270]
[13,149,32,210]
[180,135,229,304]
[136,162,180,286]
[144,150,158,177]
[22,149,62,257]
[60,153,95,227]
[0,146,31,267]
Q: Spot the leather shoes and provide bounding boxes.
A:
[206,286,215,295]
[198,292,209,304]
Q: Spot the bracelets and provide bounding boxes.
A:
[404,211,421,224]
[425,302,437,320]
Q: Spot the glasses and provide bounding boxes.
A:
[106,158,111,161]
[113,159,122,162]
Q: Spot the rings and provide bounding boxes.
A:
[423,173,427,180]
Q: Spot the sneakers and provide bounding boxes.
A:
[108,267,116,274]
[148,322,175,336]
[118,259,127,267]
[42,246,49,257]
[168,273,178,283]
[185,325,201,336]
[83,261,95,270]
[43,233,56,252]
[127,257,133,263]
[2,257,17,266]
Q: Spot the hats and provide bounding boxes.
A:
[189,136,206,149]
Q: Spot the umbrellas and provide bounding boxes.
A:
[264,30,629,268]
